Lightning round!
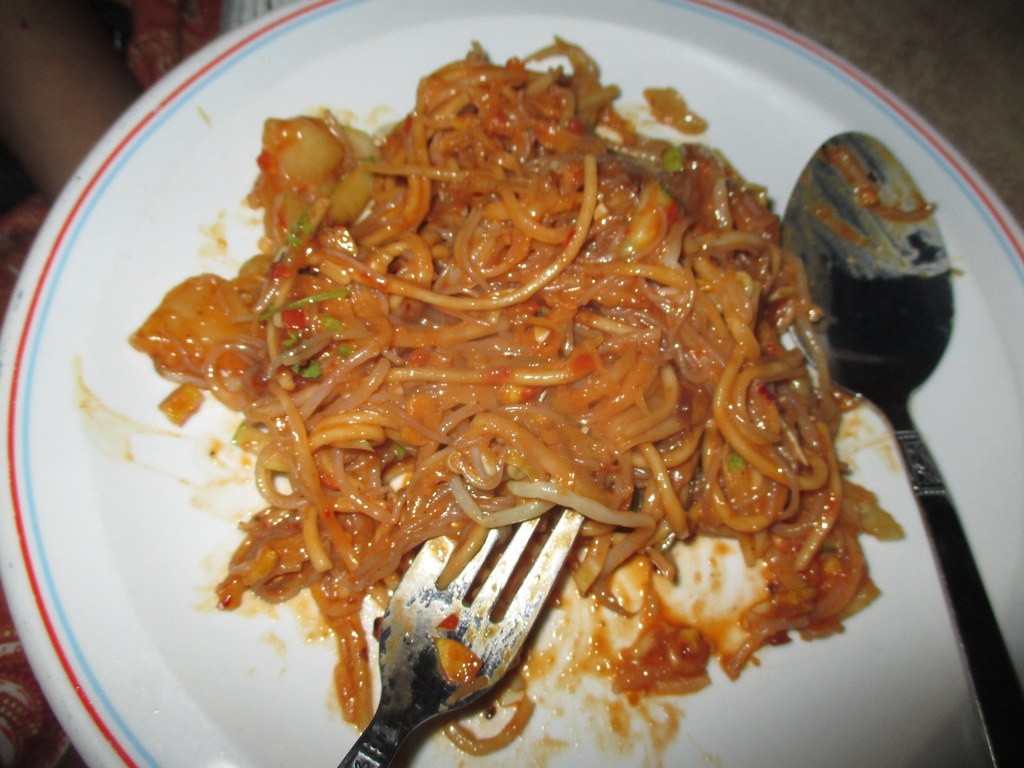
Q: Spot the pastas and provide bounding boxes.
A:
[128,33,909,761]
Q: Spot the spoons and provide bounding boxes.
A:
[784,130,1023,766]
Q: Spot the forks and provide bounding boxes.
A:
[332,472,586,766]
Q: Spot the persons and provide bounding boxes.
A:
[1,0,145,203]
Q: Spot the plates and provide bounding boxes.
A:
[7,0,1023,768]
